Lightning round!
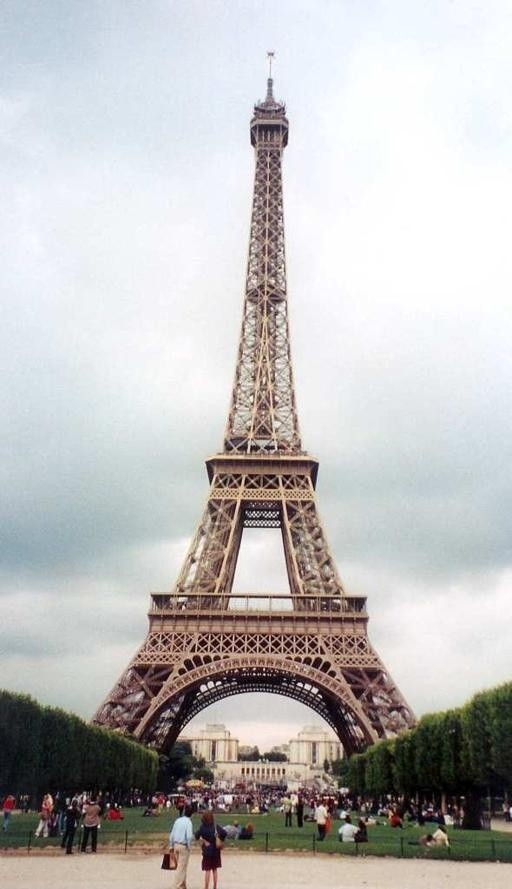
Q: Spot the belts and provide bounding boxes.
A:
[174,841,187,846]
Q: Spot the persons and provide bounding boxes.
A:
[169,804,195,889]
[509,804,512,821]
[195,810,227,889]
[502,800,510,821]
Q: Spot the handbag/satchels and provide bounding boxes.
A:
[160,848,178,870]
[213,823,226,850]
